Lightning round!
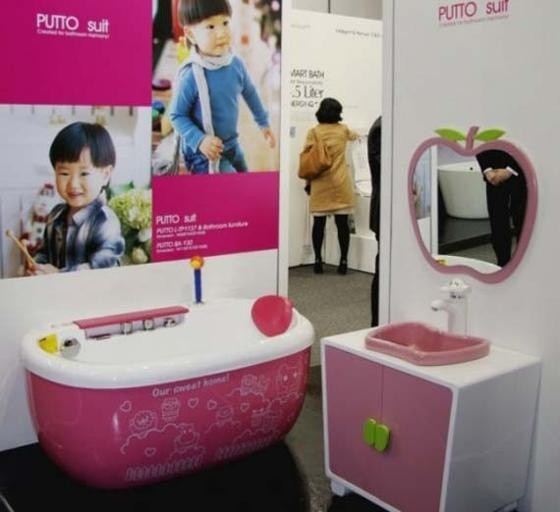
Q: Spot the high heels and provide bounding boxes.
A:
[314,258,323,273]
[338,259,347,274]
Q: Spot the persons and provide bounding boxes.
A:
[25,122,124,276]
[475,150,527,266]
[169,0,276,175]
[303,97,362,274]
[369,114,380,325]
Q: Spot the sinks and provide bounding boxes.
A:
[366,320,490,365]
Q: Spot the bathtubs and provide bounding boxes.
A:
[437,161,490,220]
[21,298,314,489]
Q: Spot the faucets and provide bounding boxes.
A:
[430,280,472,333]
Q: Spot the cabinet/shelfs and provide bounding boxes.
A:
[320,338,541,512]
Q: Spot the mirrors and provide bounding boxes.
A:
[407,135,537,282]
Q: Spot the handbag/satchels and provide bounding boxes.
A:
[298,142,331,180]
